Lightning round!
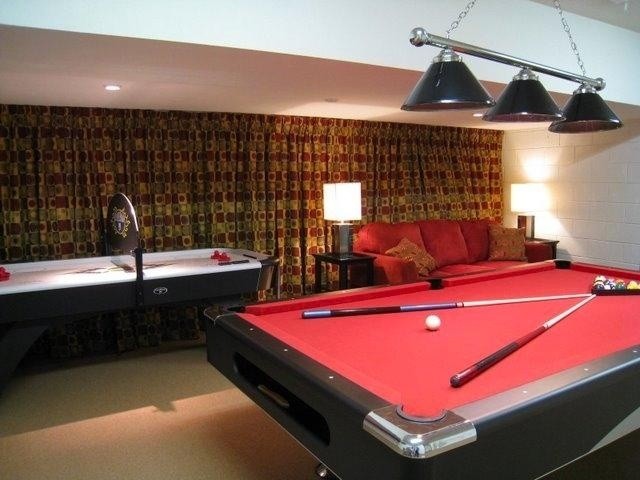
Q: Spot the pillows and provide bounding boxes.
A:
[385,236,440,277]
[486,224,527,262]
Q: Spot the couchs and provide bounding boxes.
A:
[351,220,560,285]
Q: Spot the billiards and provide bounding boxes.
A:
[425,314,442,331]
[592,274,640,290]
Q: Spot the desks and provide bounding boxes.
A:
[1,246,282,387]
[313,252,377,291]
[204,260,640,480]
[524,236,560,264]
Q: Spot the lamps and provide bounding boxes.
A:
[399,0,624,136]
[509,182,554,240]
[320,181,363,253]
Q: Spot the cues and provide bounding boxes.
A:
[301,294,595,319]
[450,295,600,389]
